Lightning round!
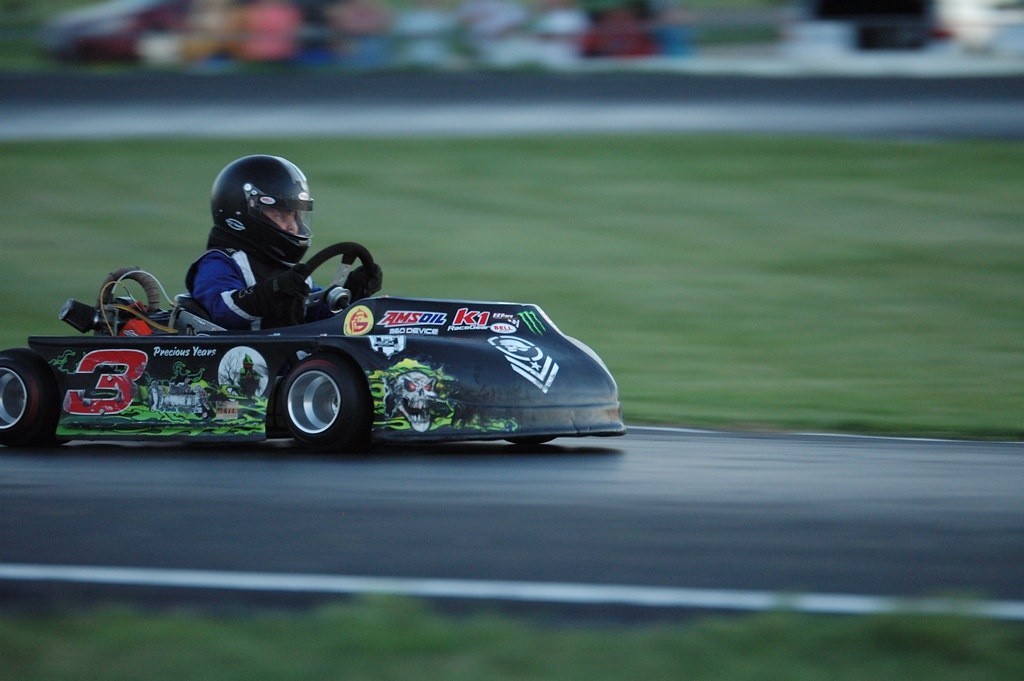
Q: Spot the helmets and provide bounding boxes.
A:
[207,154,314,264]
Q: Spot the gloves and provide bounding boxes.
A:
[254,263,309,302]
[342,263,382,305]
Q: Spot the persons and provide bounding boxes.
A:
[185,155,382,332]
